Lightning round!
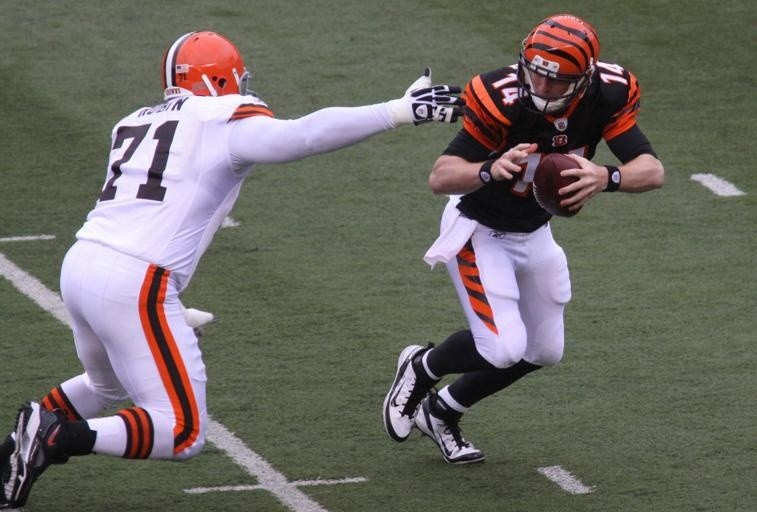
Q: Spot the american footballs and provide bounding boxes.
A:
[533,152,584,217]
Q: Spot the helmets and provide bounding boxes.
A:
[516,13,600,115]
[163,31,250,102]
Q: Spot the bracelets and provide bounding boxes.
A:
[479,158,496,185]
[603,164,621,193]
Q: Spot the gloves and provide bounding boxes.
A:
[407,67,466,127]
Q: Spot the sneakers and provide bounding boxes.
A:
[2,401,71,509]
[382,344,436,443]
[414,394,485,464]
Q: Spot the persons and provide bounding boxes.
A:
[0,31,465,509]
[382,14,666,465]
